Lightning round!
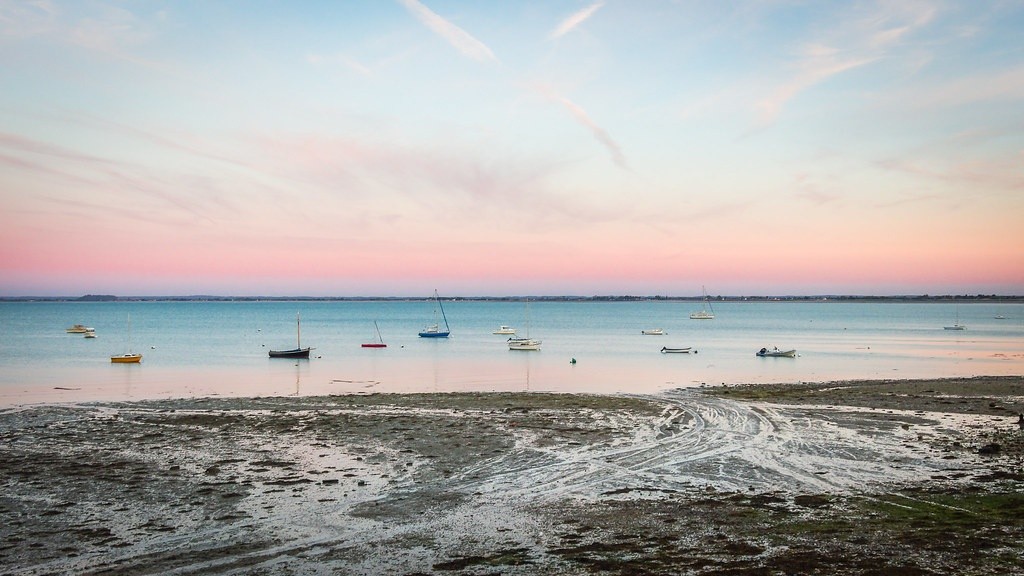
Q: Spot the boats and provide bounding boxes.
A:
[418,289,451,338]
[689,285,715,320]
[506,299,543,350]
[755,347,797,356]
[660,345,693,353]
[944,326,966,331]
[267,311,311,358]
[64,322,97,338]
[109,312,143,363]
[360,320,388,347]
[641,328,665,335]
[492,325,515,335]
